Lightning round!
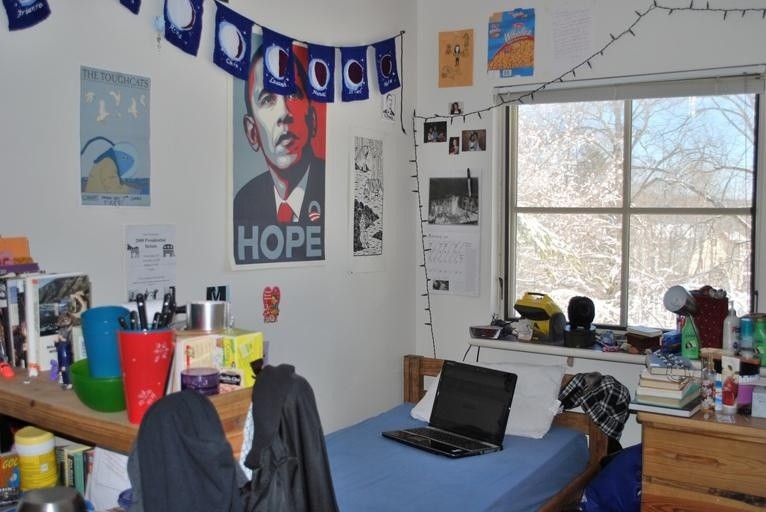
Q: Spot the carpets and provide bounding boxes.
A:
[277,200,295,222]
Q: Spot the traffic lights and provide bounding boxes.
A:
[409,357,570,440]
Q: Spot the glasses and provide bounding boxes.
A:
[662,284,705,359]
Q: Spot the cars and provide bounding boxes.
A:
[181,368,219,399]
[115,325,178,428]
[80,306,136,379]
[190,301,224,332]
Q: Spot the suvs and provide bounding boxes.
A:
[81,305,130,378]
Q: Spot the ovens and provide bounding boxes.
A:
[117,347,613,510]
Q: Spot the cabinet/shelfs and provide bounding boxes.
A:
[700,352,716,413]
[740,319,756,359]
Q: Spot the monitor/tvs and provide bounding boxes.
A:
[628,350,703,418]
[0,235,92,373]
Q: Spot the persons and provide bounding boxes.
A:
[427,125,445,142]
[449,138,459,154]
[453,44,463,65]
[382,94,395,120]
[450,102,461,115]
[233,43,327,262]
[468,132,483,151]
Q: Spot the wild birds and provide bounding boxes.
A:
[382,360,517,458]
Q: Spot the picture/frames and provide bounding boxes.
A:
[0,363,256,470]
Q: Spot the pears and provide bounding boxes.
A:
[754,319,765,366]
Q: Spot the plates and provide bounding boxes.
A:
[635,409,766,511]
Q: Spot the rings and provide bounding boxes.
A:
[466,168,472,198]
[118,293,174,331]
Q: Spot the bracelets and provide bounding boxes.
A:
[69,357,127,414]
